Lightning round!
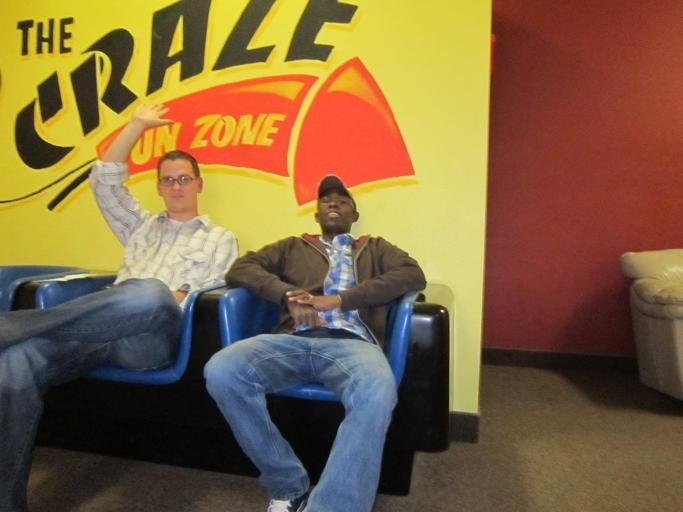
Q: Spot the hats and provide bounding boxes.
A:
[317,175,357,210]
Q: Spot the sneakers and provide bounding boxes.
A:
[267,493,309,512]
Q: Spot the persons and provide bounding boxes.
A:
[0,102,240,511]
[202,175,427,511]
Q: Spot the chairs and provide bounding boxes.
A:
[619,247,682,399]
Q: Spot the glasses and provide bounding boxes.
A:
[319,195,354,210]
[159,175,196,186]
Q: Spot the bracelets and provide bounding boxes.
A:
[337,294,342,303]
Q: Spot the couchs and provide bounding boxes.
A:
[0,266,450,496]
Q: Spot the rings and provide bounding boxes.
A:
[309,295,313,300]
